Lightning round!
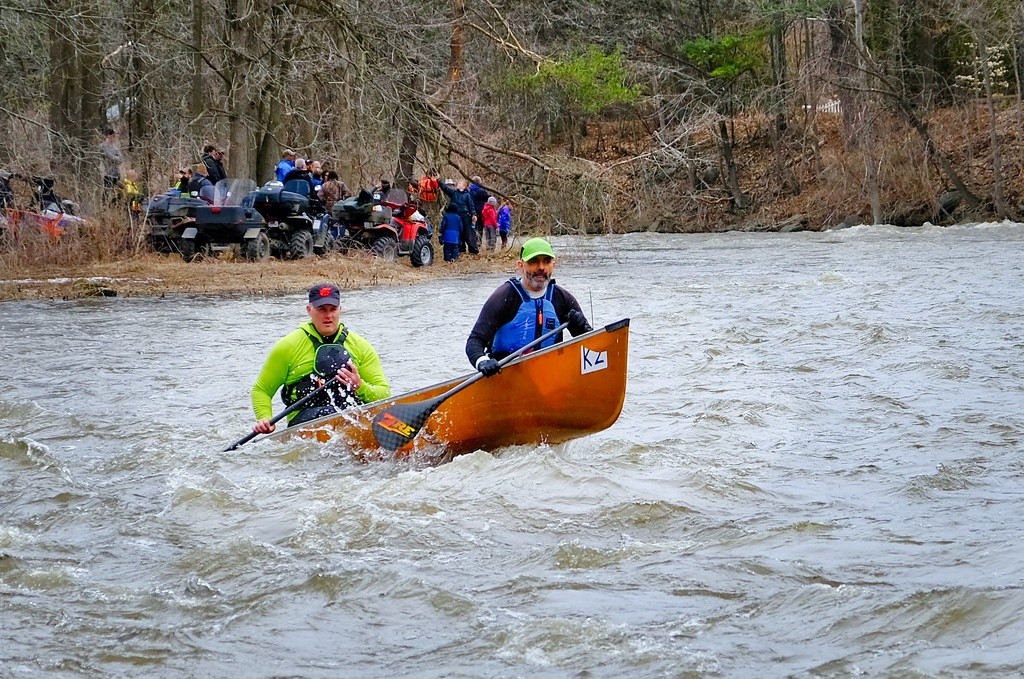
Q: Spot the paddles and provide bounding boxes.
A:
[372,320,571,453]
[216,368,350,453]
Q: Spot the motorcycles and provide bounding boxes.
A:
[139,188,211,252]
[181,178,348,262]
[332,188,434,267]
[0,170,91,237]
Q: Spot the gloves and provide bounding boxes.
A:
[478,359,502,378]
[564,309,586,334]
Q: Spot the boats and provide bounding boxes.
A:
[251,318,630,462]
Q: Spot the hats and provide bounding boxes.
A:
[444,179,456,186]
[180,168,193,174]
[309,283,340,308]
[488,196,496,206]
[449,203,457,211]
[282,149,295,156]
[520,237,555,262]
[305,160,313,163]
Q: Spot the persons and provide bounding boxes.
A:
[495,198,512,248]
[252,283,391,434]
[420,167,438,206]
[172,164,220,199]
[200,146,231,192]
[436,177,479,254]
[274,149,352,215]
[468,176,491,249]
[439,203,464,263]
[100,129,122,186]
[370,179,390,197]
[123,170,140,223]
[482,197,499,251]
[465,237,594,378]
